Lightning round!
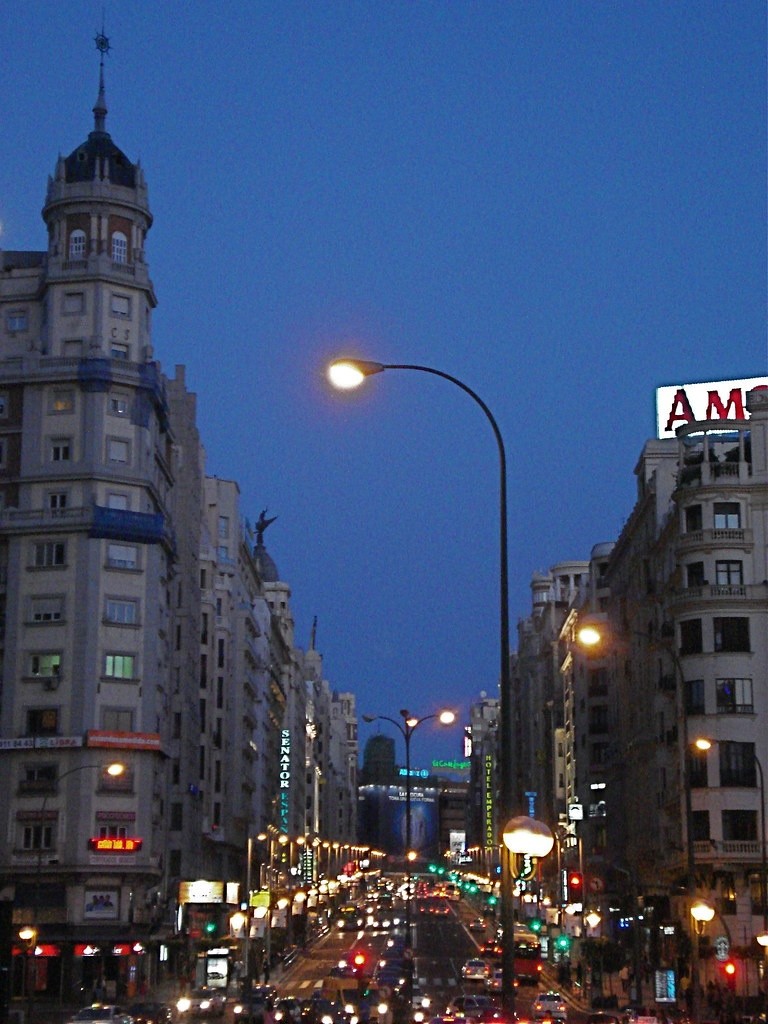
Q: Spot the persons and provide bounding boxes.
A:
[90,894,113,913]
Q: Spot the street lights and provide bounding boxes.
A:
[27,762,124,1024]
[333,842,340,872]
[695,738,767,934]
[360,708,455,1015]
[322,842,330,879]
[327,355,516,1023]
[312,841,320,865]
[289,836,307,869]
[466,847,480,871]
[244,834,267,977]
[340,844,369,866]
[577,623,702,1024]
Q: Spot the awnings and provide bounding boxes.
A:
[0,924,184,949]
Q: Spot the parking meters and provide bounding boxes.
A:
[270,834,288,885]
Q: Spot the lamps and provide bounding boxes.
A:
[49,860,59,864]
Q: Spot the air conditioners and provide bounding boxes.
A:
[43,678,58,690]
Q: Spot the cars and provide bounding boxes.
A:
[62,1002,136,1024]
[129,1000,179,1024]
[232,874,567,1024]
[586,1009,630,1024]
[178,987,228,1019]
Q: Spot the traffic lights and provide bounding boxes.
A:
[725,961,736,990]
[568,872,583,904]
